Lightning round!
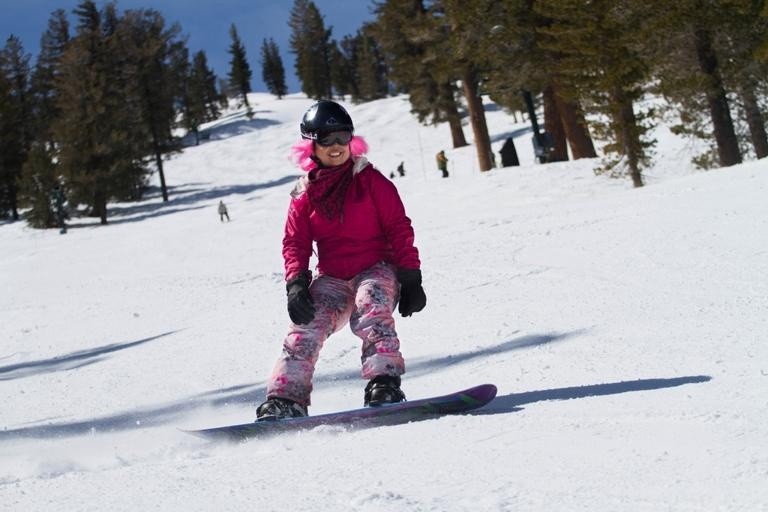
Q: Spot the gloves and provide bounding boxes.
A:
[399,269,426,317]
[286,270,316,325]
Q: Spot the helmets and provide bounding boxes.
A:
[300,100,354,141]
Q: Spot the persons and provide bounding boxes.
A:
[390,171,394,179]
[397,161,406,179]
[256,100,427,421]
[218,200,230,222]
[436,150,449,177]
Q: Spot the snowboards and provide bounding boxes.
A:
[175,384,497,441]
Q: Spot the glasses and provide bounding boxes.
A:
[317,131,352,146]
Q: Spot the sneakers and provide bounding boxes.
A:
[256,397,308,422]
[364,377,407,408]
